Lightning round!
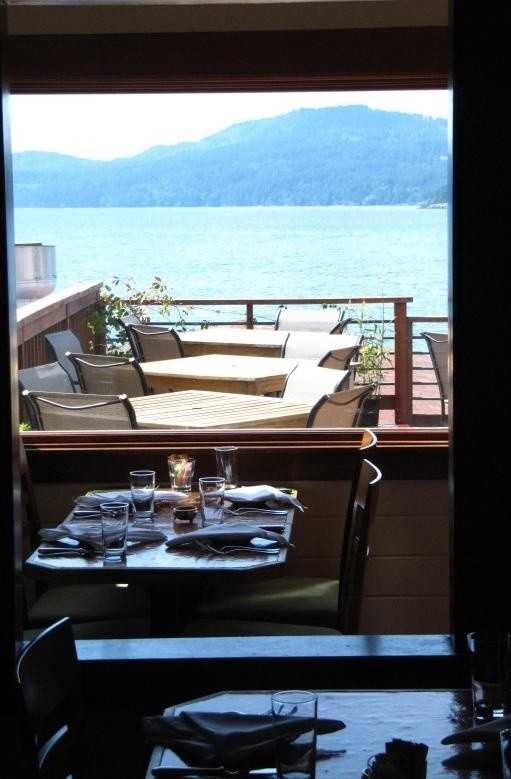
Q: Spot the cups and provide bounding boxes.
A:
[472,718,507,754]
[35,446,294,563]
[463,630,511,716]
[272,688,317,779]
